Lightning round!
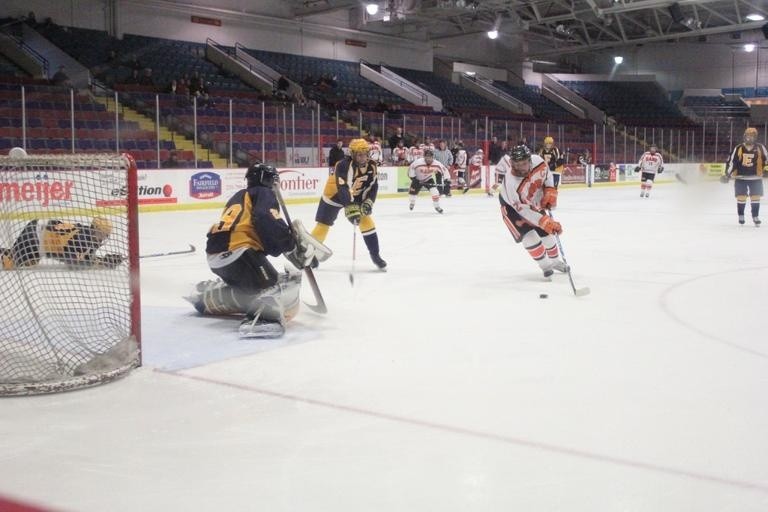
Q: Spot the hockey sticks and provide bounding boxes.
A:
[419,179,481,188]
[124,245,195,261]
[349,220,357,284]
[276,190,328,314]
[550,206,578,297]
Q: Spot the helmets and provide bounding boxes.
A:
[743,128,757,143]
[93,217,110,243]
[350,138,371,154]
[510,146,532,176]
[545,136,554,146]
[246,163,279,188]
[424,149,435,158]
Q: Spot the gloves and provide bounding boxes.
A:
[345,204,361,225]
[444,181,451,195]
[361,199,373,214]
[104,253,122,267]
[537,216,562,233]
[541,187,558,208]
[721,176,730,184]
[658,168,664,173]
[635,167,641,172]
[410,176,419,189]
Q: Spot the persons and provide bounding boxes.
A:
[633,143,664,198]
[29,10,593,213]
[498,143,570,276]
[0,217,121,272]
[721,127,768,224]
[192,161,333,339]
[310,137,388,268]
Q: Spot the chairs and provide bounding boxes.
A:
[0,15,768,168]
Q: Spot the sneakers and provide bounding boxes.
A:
[371,253,386,268]
[436,207,443,212]
[640,193,650,197]
[239,320,284,337]
[543,263,570,277]
[739,215,762,224]
[410,205,413,209]
